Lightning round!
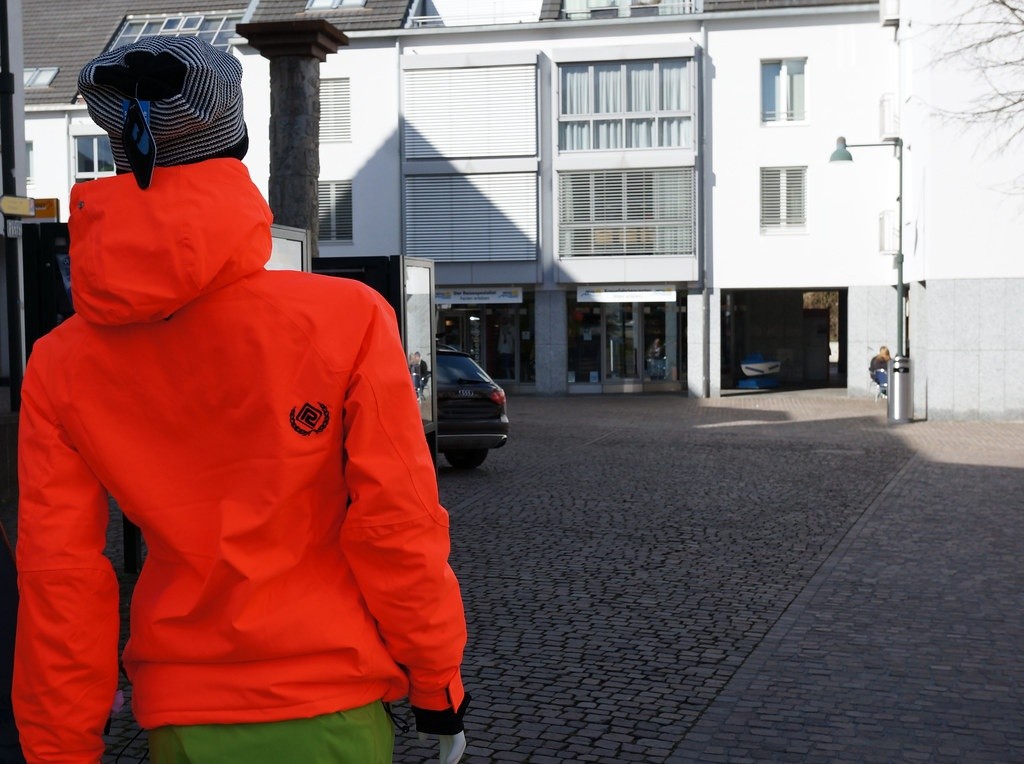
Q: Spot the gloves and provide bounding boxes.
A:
[418,731,466,764]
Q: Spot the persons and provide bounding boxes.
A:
[408,351,429,400]
[496,315,516,380]
[9,32,473,764]
[868,346,893,399]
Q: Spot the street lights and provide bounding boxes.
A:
[828,135,906,359]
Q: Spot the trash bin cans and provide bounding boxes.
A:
[888,356,916,423]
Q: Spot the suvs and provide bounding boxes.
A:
[434,341,510,471]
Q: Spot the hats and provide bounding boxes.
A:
[76,35,251,174]
